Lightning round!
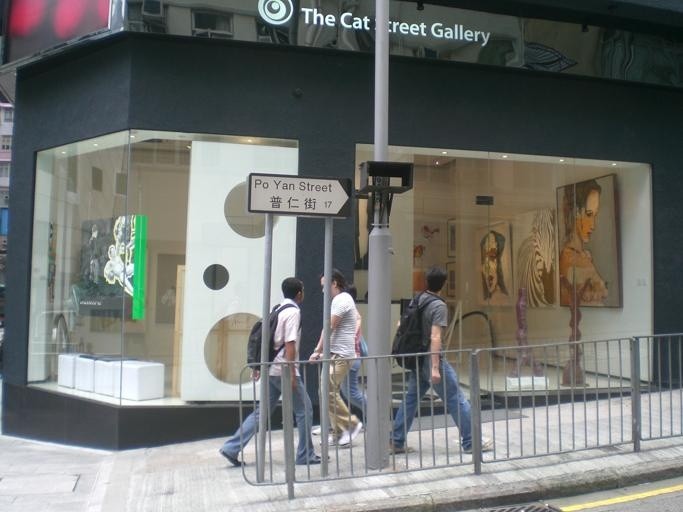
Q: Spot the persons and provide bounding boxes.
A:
[79,215,135,300]
[310,283,366,436]
[556,178,612,309]
[478,230,511,305]
[218,277,320,467]
[305,268,363,448]
[387,265,493,456]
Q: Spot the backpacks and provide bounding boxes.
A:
[390,288,444,370]
[245,301,302,373]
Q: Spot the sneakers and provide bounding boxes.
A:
[387,441,415,455]
[461,437,495,453]
[311,421,362,447]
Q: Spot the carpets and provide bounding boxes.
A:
[391,409,529,432]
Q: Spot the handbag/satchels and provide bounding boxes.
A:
[358,334,369,358]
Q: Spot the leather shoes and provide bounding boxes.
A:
[217,447,246,466]
[294,453,330,465]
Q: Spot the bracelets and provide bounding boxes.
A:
[312,348,320,353]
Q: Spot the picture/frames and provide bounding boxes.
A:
[446,300,455,322]
[447,218,456,258]
[474,221,514,308]
[446,262,456,297]
